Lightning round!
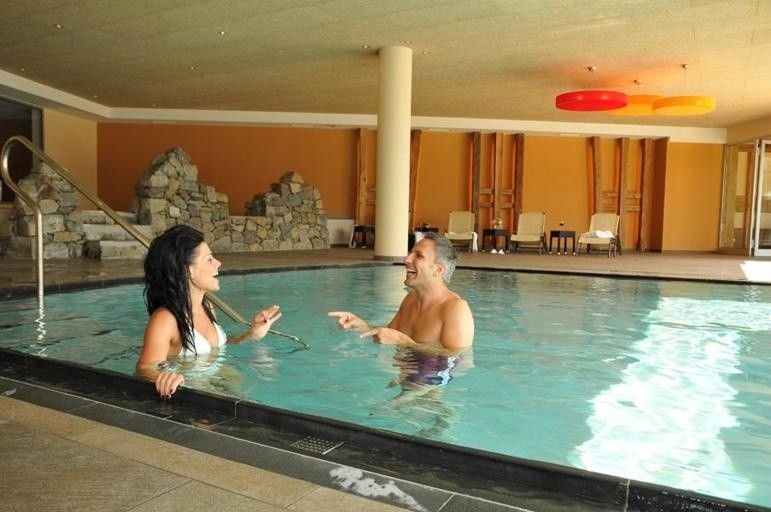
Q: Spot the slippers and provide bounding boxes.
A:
[490,248,506,255]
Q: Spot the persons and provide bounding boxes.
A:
[327,232,475,350]
[130,224,283,402]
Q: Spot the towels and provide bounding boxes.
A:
[581,231,614,238]
[471,232,478,253]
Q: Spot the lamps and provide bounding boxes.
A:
[653,64,716,116]
[606,81,664,116]
[555,67,627,111]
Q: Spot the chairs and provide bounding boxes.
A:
[510,212,547,255]
[575,213,621,257]
[443,210,475,253]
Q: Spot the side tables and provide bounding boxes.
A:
[482,229,509,253]
[549,230,575,256]
[415,227,438,233]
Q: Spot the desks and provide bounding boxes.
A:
[352,225,375,249]
